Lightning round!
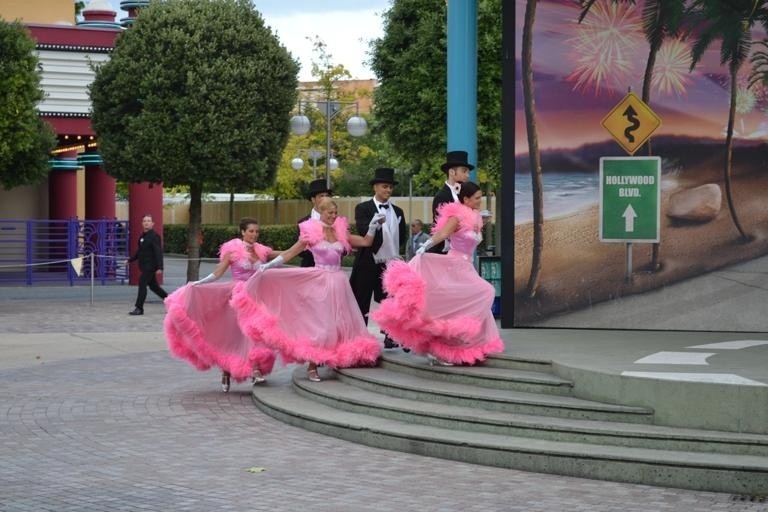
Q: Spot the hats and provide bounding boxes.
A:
[441,151,474,173]
[369,168,400,185]
[308,179,332,201]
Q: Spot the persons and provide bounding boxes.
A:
[405,218,430,260]
[414,180,505,368]
[121,214,171,316]
[476,210,495,257]
[295,177,342,267]
[425,151,476,256]
[347,167,407,349]
[187,218,295,393]
[258,196,387,383]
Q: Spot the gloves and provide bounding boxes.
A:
[416,238,434,255]
[191,273,218,287]
[367,214,386,237]
[259,254,285,272]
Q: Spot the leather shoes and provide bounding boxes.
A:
[222,374,230,393]
[306,366,321,382]
[129,306,143,315]
[250,370,265,385]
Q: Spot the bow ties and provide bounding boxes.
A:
[379,204,389,209]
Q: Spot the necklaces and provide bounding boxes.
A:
[320,221,333,229]
[243,241,254,248]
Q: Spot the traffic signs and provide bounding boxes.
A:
[600,157,661,244]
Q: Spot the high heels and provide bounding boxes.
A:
[427,352,455,366]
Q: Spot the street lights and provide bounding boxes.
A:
[291,95,367,196]
[291,148,338,183]
